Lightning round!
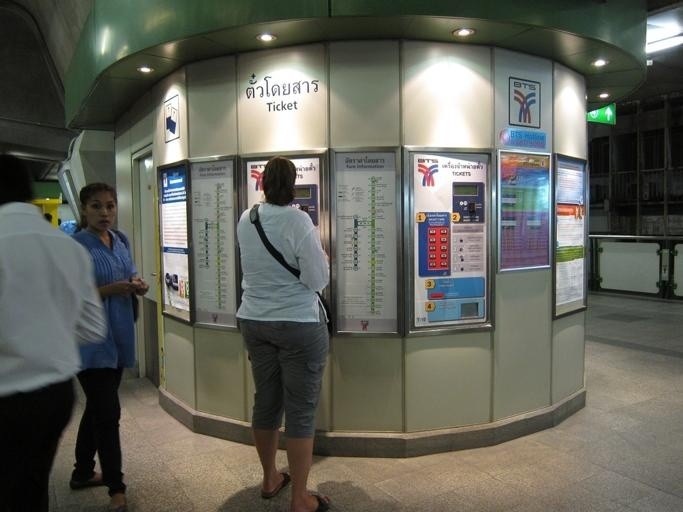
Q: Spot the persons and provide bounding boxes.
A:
[0,153,110,511]
[68,182,151,511]
[230,156,333,511]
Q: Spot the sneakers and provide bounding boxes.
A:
[69,471,106,489]
[95,498,128,512]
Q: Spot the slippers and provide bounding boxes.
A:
[261,472,291,500]
[308,493,331,512]
